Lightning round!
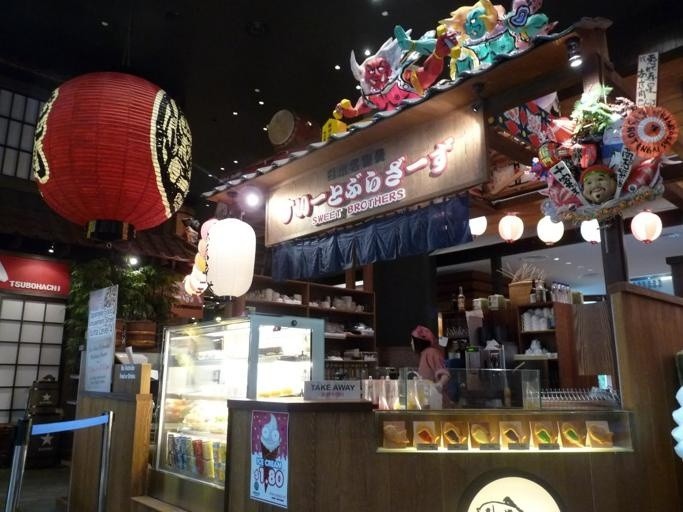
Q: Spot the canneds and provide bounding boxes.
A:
[168,435,227,482]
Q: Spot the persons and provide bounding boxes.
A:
[601,119,625,167]
[581,166,617,203]
[411,325,456,409]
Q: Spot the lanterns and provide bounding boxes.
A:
[205,217,256,302]
[581,219,602,244]
[631,208,662,245]
[469,216,487,238]
[498,214,524,244]
[537,216,564,246]
[33,72,193,242]
[183,218,219,296]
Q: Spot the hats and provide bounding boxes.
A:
[411,325,434,342]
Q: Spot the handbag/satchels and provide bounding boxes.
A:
[446,375,460,402]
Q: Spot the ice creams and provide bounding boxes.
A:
[260,413,281,493]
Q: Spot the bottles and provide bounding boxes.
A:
[529,278,572,305]
[325,363,369,380]
[457,286,466,311]
[503,381,513,408]
[367,375,431,412]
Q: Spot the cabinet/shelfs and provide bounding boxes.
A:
[144,314,325,510]
[234,275,375,380]
[517,299,575,397]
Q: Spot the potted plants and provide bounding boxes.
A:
[62,257,182,376]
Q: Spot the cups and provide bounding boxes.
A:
[245,289,303,305]
[520,307,554,334]
[524,340,558,358]
[309,295,365,313]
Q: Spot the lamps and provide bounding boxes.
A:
[562,35,585,70]
